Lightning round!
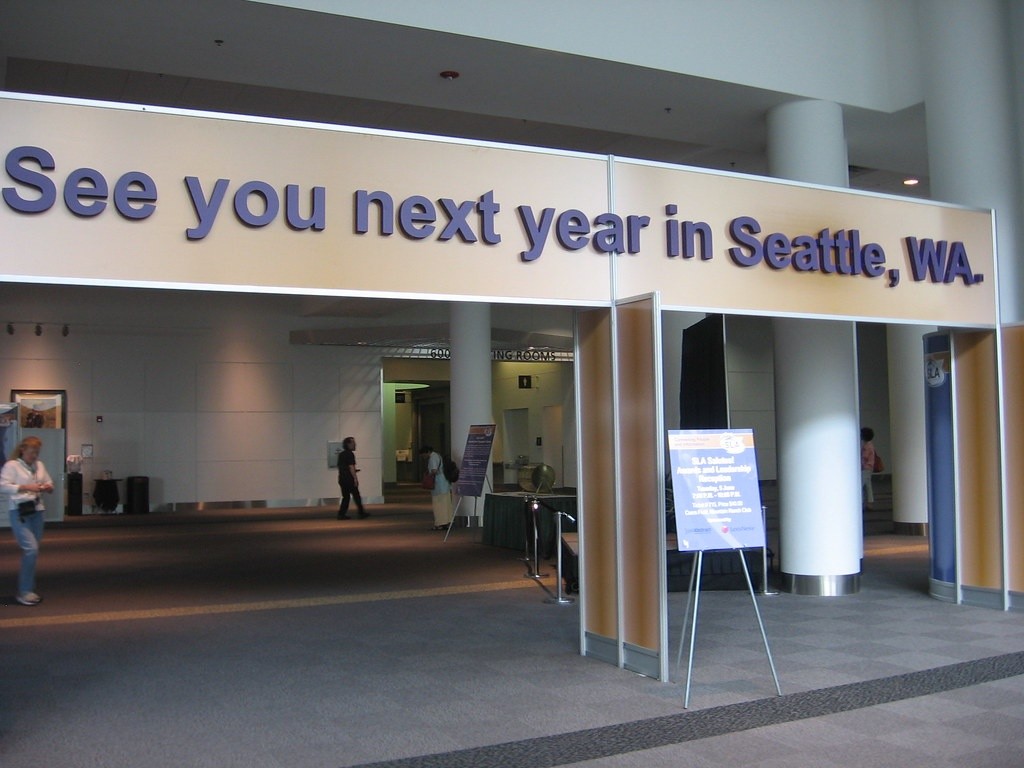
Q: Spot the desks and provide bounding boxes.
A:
[484,491,578,559]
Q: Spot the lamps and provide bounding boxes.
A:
[2,322,72,336]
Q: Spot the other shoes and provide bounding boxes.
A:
[16,591,41,606]
[337,516,353,520]
[357,512,370,519]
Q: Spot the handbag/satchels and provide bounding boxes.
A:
[18,501,35,517]
[422,475,434,489]
[872,450,883,473]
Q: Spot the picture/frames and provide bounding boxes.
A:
[11,389,66,472]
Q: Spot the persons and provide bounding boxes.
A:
[420,445,455,531]
[0,435,55,605]
[860,427,875,511]
[337,437,371,520]
[24,404,44,428]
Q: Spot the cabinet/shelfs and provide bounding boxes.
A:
[0,421,64,528]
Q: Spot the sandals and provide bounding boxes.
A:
[430,524,449,531]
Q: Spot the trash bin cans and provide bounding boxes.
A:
[127,476,149,513]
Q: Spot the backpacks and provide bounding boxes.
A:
[435,451,459,483]
